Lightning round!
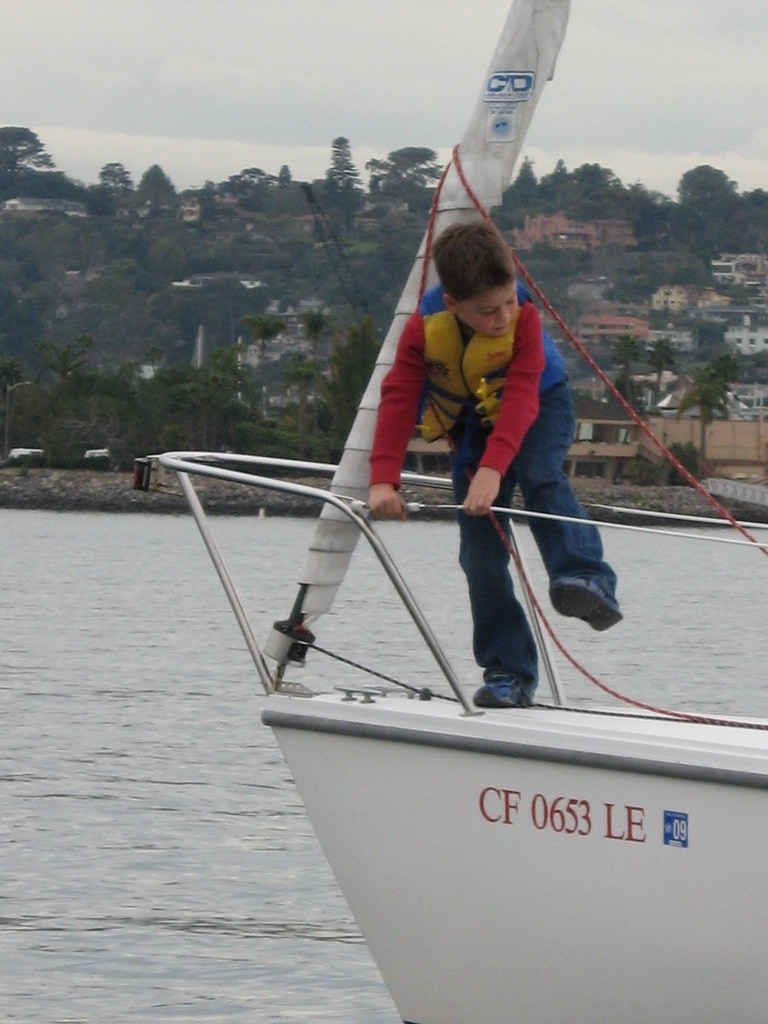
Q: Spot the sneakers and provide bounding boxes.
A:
[549,577,623,632]
[472,670,532,708]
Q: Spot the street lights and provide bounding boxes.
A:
[3,379,33,460]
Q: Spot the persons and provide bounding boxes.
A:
[368,220,623,708]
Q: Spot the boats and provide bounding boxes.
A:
[155,3,767,1024]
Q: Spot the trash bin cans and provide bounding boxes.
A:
[132,458,151,491]
